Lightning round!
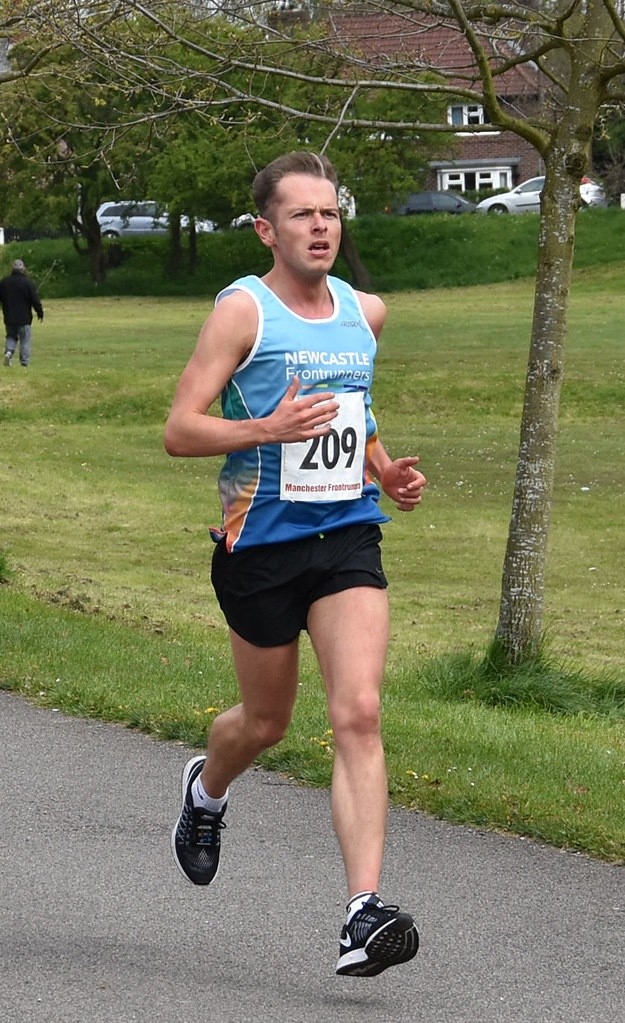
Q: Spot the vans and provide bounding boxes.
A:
[230,184,357,231]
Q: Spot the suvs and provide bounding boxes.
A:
[72,199,223,241]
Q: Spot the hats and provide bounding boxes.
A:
[581,177,591,184]
[12,259,26,274]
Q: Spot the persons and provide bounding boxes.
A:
[165,152,429,982]
[0,259,44,367]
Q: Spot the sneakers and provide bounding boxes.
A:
[21,363,27,366]
[4,352,12,369]
[335,902,420,977]
[170,755,228,886]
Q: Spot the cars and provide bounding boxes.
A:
[476,174,607,217]
[396,189,478,216]
